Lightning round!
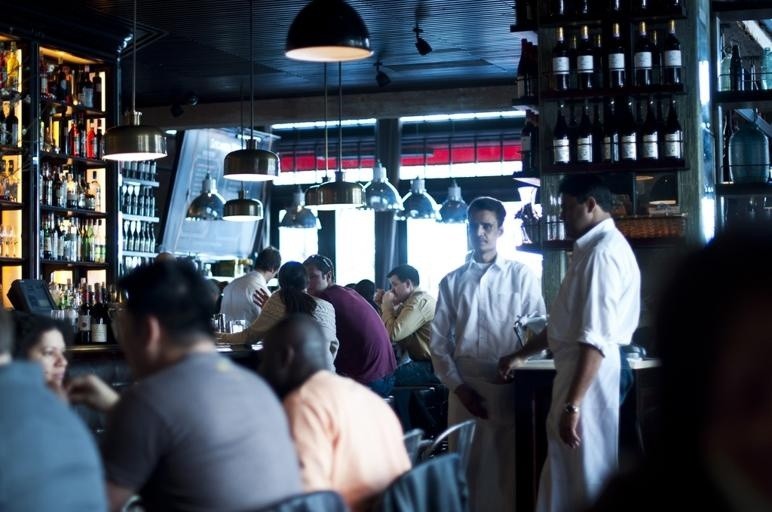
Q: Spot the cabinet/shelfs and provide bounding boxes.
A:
[0,35,110,314]
[120,176,161,259]
[711,87,771,195]
[508,10,688,253]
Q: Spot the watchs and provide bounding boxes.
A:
[561,404,580,414]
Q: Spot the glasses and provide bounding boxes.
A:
[108,308,131,320]
[309,255,332,272]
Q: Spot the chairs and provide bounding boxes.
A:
[369,454,472,512]
[380,380,479,470]
[263,490,345,511]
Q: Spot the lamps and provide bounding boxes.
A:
[278,128,320,231]
[222,132,263,223]
[187,129,225,223]
[303,61,369,212]
[225,44,277,180]
[372,21,433,90]
[98,3,168,164]
[281,0,375,64]
[353,118,469,223]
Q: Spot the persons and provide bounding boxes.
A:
[496,169,642,512]
[66,252,302,511]
[262,312,411,511]
[215,247,439,399]
[593,212,772,511]
[429,196,548,511]
[1,313,109,511]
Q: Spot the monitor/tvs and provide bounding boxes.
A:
[7,279,59,314]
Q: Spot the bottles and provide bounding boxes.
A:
[761,46,772,89]
[120,158,157,253]
[552,16,680,86]
[723,110,736,184]
[49,275,123,345]
[516,1,535,171]
[730,42,747,91]
[722,51,735,91]
[551,100,688,170]
[0,41,101,267]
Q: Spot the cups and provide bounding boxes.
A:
[229,318,245,334]
